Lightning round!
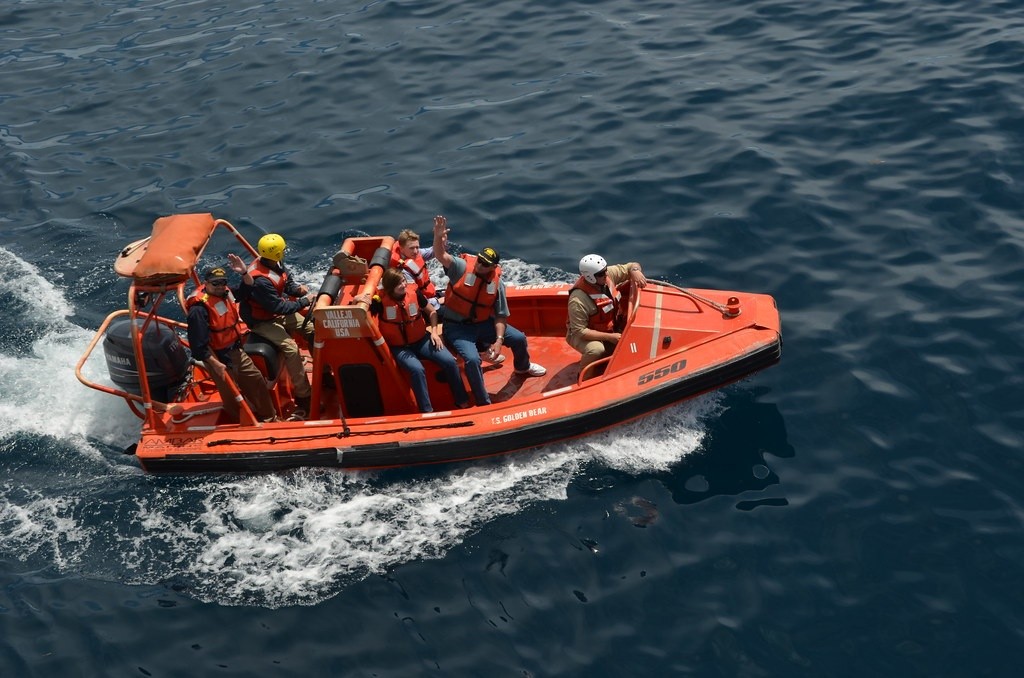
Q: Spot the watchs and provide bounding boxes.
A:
[629,267,639,271]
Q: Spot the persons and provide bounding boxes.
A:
[566,254,648,381]
[348,268,472,413]
[391,229,506,365]
[186,253,287,423]
[240,234,335,414]
[433,216,547,408]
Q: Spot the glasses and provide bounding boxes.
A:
[208,280,227,286]
[477,258,494,268]
[594,268,608,277]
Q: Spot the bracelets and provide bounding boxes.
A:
[496,336,505,341]
[243,271,248,275]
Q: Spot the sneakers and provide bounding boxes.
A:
[515,362,547,377]
[478,350,506,365]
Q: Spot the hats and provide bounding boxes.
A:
[477,248,499,265]
[204,266,229,282]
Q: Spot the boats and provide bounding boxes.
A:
[74,210,787,479]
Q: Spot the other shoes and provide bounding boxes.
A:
[460,400,472,409]
[294,395,326,414]
[322,375,337,388]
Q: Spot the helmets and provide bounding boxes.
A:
[258,233,286,262]
[579,254,607,284]
